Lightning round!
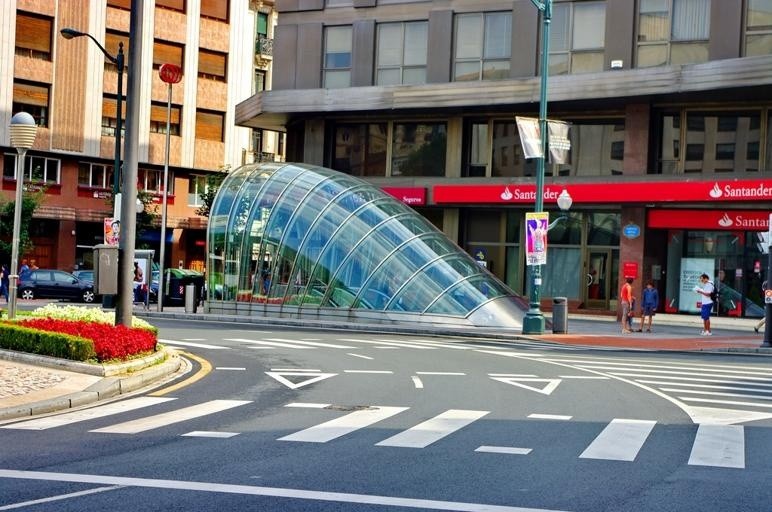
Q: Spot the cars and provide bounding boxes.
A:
[20,267,100,302]
[74,269,158,306]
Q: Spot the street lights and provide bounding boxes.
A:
[7,111,37,319]
[522,1,572,333]
[60,28,124,197]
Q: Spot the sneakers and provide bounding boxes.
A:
[701,330,712,335]
[622,328,651,334]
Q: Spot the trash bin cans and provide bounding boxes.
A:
[185,285,196,314]
[552,297,568,334]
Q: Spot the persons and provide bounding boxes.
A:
[753,281,767,334]
[134,262,143,282]
[262,271,274,297]
[0,259,39,304]
[620,277,632,334]
[635,280,659,333]
[109,221,119,242]
[141,283,148,306]
[695,273,715,336]
[626,293,635,331]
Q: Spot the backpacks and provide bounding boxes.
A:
[707,282,718,303]
